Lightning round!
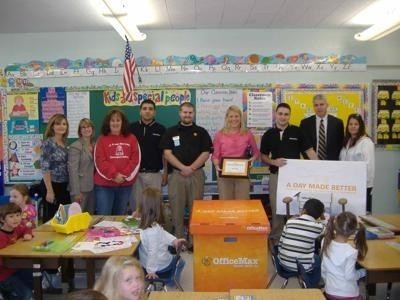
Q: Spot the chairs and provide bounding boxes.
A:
[266,245,302,290]
[293,256,324,291]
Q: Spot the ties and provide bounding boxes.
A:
[318,120,327,160]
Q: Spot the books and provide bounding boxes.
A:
[364,226,394,240]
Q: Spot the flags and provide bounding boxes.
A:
[122,38,138,93]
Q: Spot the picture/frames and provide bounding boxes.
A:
[218,156,252,179]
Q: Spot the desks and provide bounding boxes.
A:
[359,213,399,235]
[232,288,328,300]
[0,205,189,299]
[146,288,231,300]
[353,235,400,297]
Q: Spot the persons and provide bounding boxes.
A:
[260,94,374,239]
[66,117,97,215]
[137,185,188,291]
[8,183,63,295]
[159,101,213,254]
[94,254,160,299]
[320,211,368,299]
[128,100,168,227]
[211,104,259,200]
[40,113,72,223]
[0,202,35,299]
[277,198,329,288]
[93,110,141,215]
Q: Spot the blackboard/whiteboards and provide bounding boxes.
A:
[372,79,400,149]
[2,83,373,185]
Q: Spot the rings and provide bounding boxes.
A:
[80,199,82,202]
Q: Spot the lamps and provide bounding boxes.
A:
[354,14,400,42]
[104,11,148,43]
